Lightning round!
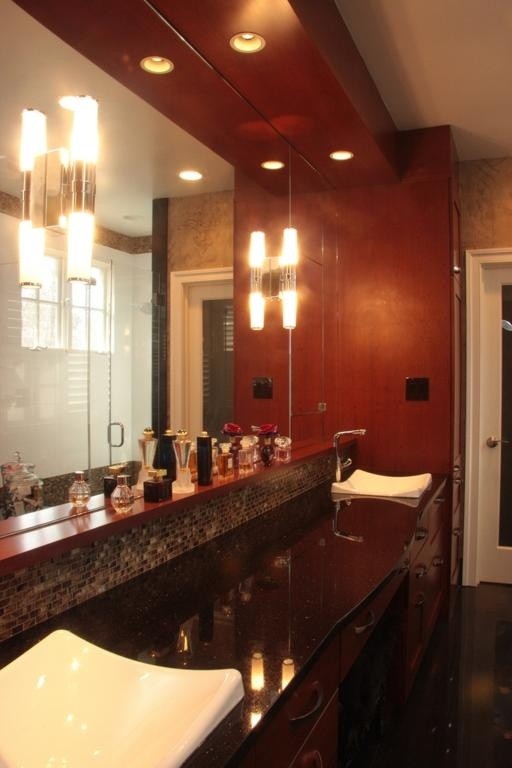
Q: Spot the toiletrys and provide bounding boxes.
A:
[103,464,128,498]
[110,422,292,515]
[69,470,92,507]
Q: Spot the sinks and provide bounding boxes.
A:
[0,630,248,765]
[333,467,433,499]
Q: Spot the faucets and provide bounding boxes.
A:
[331,501,367,544]
[332,428,367,481]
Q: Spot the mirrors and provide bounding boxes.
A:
[2,0,339,540]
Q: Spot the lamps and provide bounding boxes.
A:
[275,228,300,331]
[48,88,102,297]
[247,231,273,334]
[11,93,44,303]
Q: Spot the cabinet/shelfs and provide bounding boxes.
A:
[237,634,338,767]
[409,483,449,689]
